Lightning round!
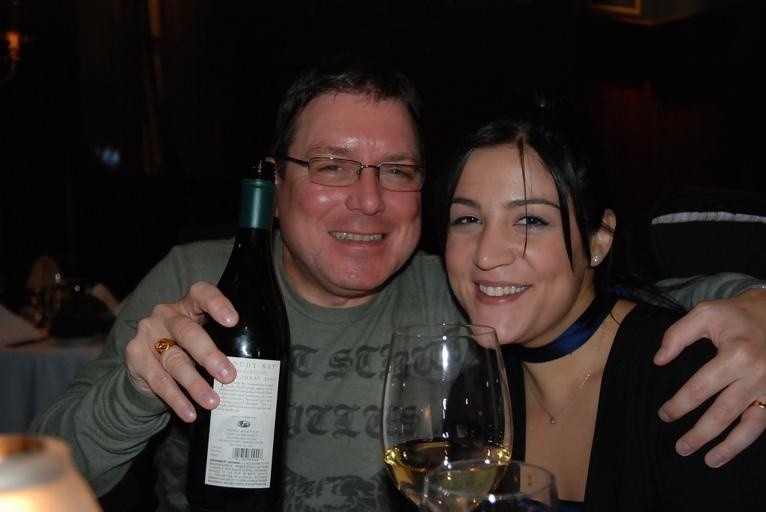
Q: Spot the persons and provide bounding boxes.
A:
[418,80,766,511]
[18,51,766,512]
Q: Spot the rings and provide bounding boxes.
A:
[753,400,766,409]
[154,337,177,354]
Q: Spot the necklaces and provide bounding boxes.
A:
[523,317,610,424]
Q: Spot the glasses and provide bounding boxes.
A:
[279,151,428,196]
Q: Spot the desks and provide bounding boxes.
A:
[0,331,109,433]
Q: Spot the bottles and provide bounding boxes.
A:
[185,161,291,512]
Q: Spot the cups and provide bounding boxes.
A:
[381,323,559,512]
